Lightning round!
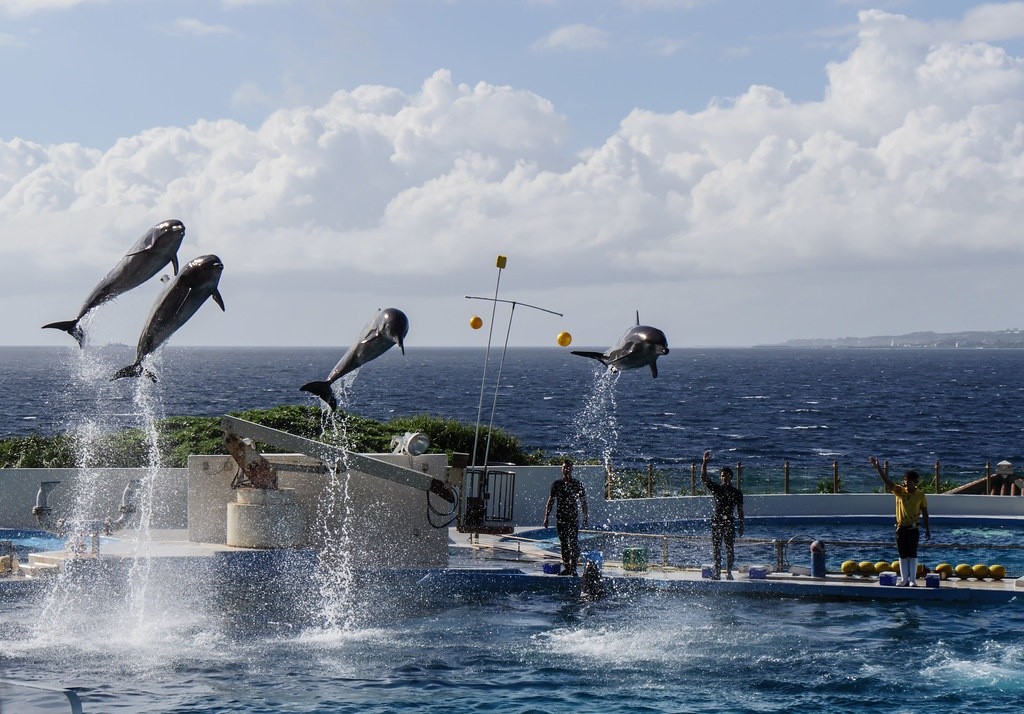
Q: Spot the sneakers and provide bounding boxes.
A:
[896,581,917,587]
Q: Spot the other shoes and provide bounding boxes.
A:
[556,569,579,577]
[711,575,721,580]
[726,574,734,580]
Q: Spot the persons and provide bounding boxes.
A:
[543,460,589,575]
[871,456,931,589]
[701,451,744,582]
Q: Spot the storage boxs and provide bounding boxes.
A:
[702,564,715,578]
[879,571,897,586]
[543,561,561,574]
[925,573,940,588]
[748,566,766,579]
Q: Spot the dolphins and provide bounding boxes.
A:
[297,306,410,412]
[570,310,670,379]
[37,219,186,350]
[109,254,225,384]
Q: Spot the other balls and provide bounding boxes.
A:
[556,331,571,347]
[841,560,1006,582]
[470,316,482,328]
